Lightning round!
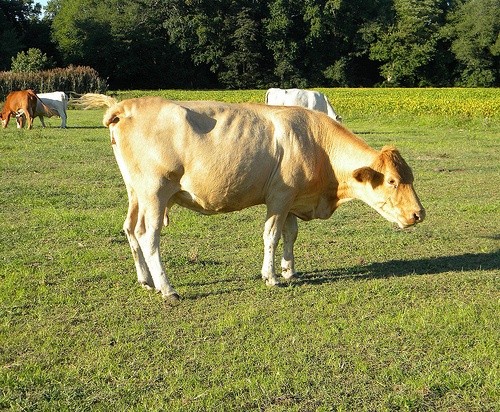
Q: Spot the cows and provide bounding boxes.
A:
[0,89,60,129]
[14,92,67,129]
[265,88,342,124]
[68,91,427,308]
